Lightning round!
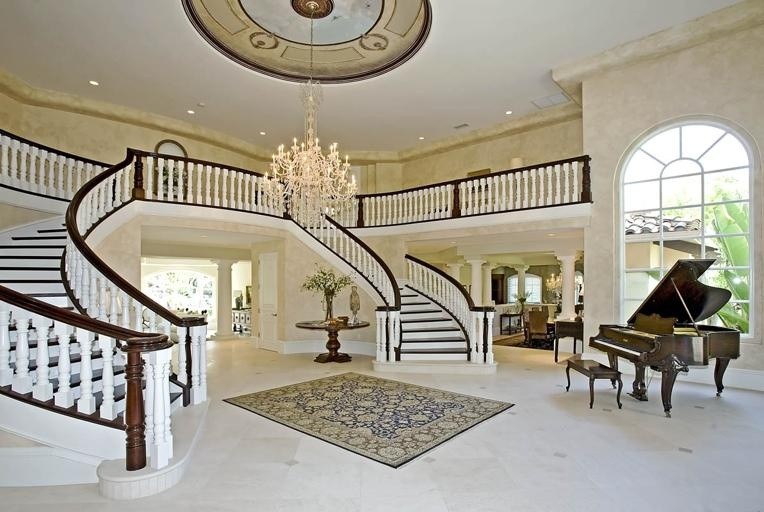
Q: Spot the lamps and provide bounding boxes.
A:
[258,9,359,229]
[544,272,562,298]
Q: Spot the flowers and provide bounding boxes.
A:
[299,261,357,313]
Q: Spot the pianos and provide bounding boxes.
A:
[589,259,740,418]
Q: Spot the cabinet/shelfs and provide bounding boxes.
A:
[232,308,251,335]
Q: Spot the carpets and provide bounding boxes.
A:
[222,371,517,470]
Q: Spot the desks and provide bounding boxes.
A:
[524,319,555,344]
[555,320,582,362]
[296,318,371,363]
[499,313,522,335]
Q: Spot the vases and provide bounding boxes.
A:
[323,293,336,323]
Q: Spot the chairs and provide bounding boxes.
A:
[529,311,554,349]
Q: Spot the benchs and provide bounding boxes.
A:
[566,360,623,409]
